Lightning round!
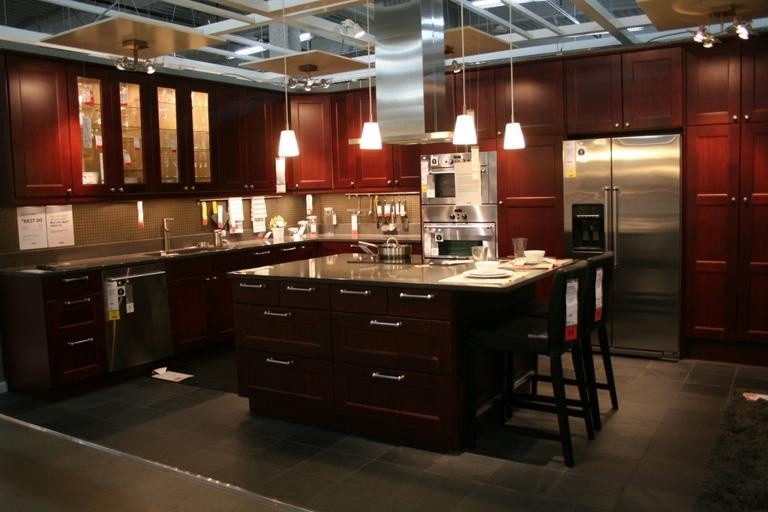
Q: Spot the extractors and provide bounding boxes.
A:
[349,0,455,150]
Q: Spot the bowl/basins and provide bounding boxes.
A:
[474,261,500,273]
[523,249,545,261]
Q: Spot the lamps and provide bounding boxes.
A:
[452,1,483,150]
[360,1,385,153]
[278,0,302,163]
[499,0,528,154]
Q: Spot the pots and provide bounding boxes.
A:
[349,238,413,265]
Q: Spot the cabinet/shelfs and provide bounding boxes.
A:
[0,42,456,214]
[330,278,475,459]
[0,239,330,434]
[454,28,768,370]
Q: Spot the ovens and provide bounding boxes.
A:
[420,152,500,262]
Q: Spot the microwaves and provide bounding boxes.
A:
[346,258,457,263]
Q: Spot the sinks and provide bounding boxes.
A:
[144,245,228,258]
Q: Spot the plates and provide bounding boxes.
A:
[462,269,515,279]
[519,260,546,264]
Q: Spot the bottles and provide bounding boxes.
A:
[215,229,224,247]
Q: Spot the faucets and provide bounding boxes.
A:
[163,218,175,250]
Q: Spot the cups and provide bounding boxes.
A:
[512,237,527,259]
[76,81,208,178]
[559,134,683,363]
[471,245,487,261]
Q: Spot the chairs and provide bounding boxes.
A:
[519,245,623,433]
[488,260,597,470]
